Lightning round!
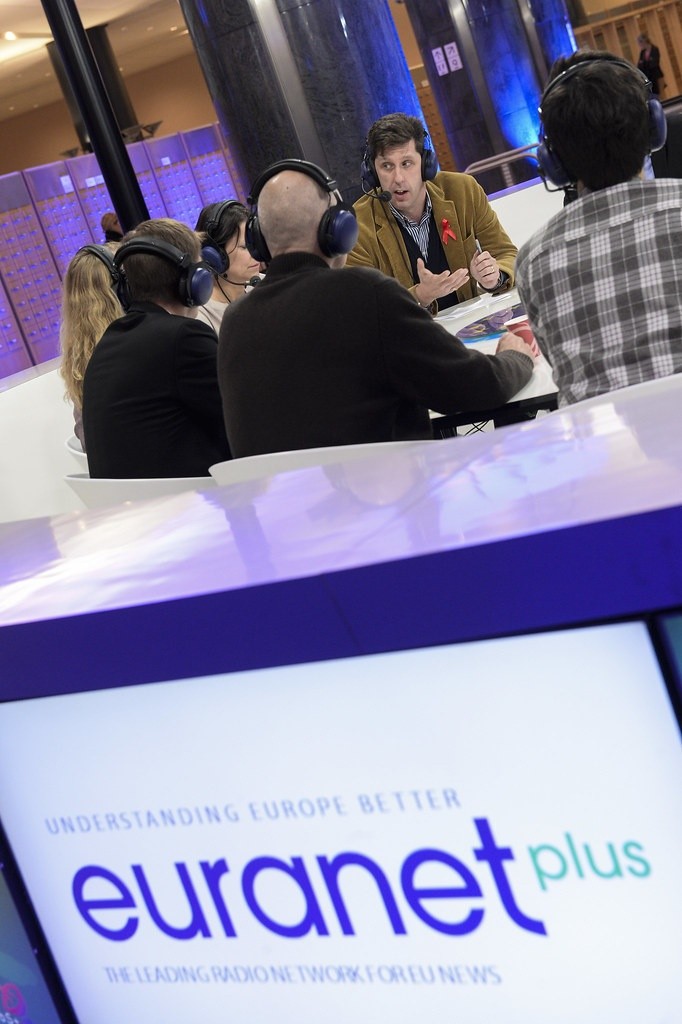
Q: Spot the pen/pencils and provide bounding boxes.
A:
[475,239,482,254]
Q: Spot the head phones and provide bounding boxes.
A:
[201,199,248,274]
[113,236,214,311]
[76,244,128,310]
[536,58,668,187]
[245,158,359,262]
[361,129,437,188]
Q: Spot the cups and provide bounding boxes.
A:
[504,314,541,361]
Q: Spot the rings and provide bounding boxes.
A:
[491,267,494,272]
[452,288,457,290]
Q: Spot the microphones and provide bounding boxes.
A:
[360,176,391,202]
[204,262,262,287]
[537,163,578,191]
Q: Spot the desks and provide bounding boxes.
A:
[420,286,563,440]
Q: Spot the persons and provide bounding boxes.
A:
[344,113,539,438]
[636,34,666,100]
[196,200,267,335]
[101,213,123,242]
[82,218,232,479]
[61,242,128,454]
[218,160,535,459]
[515,51,682,410]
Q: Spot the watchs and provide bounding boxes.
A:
[492,271,504,290]
